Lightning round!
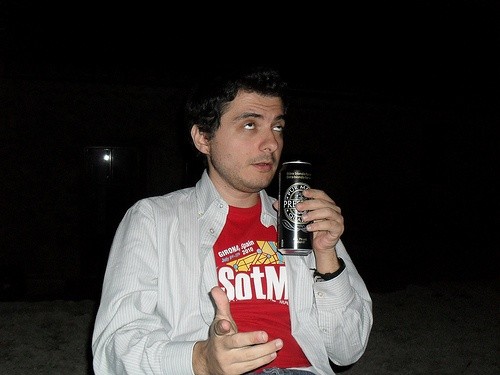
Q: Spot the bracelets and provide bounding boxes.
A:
[312,256,346,282]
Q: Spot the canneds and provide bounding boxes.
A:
[277,161,314,256]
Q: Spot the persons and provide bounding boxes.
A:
[91,64,375,375]
